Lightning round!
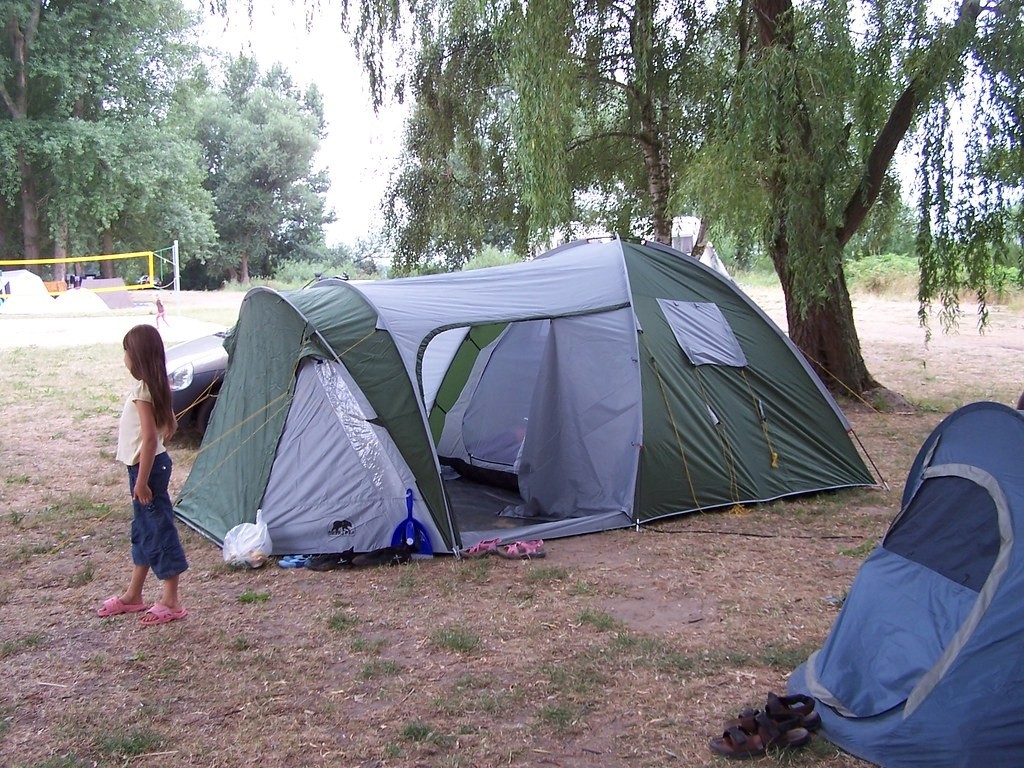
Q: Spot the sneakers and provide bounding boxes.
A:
[277,554,316,568]
[306,546,355,571]
[352,544,412,566]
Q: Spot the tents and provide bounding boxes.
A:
[172,232,892,563]
[785,401,1024,768]
[550,215,734,282]
[0,269,137,315]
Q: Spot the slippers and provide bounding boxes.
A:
[98,598,146,617]
[139,604,187,624]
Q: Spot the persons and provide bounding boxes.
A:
[97,324,189,625]
[155,299,169,329]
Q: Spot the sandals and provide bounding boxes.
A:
[459,538,546,562]
[708,694,822,756]
